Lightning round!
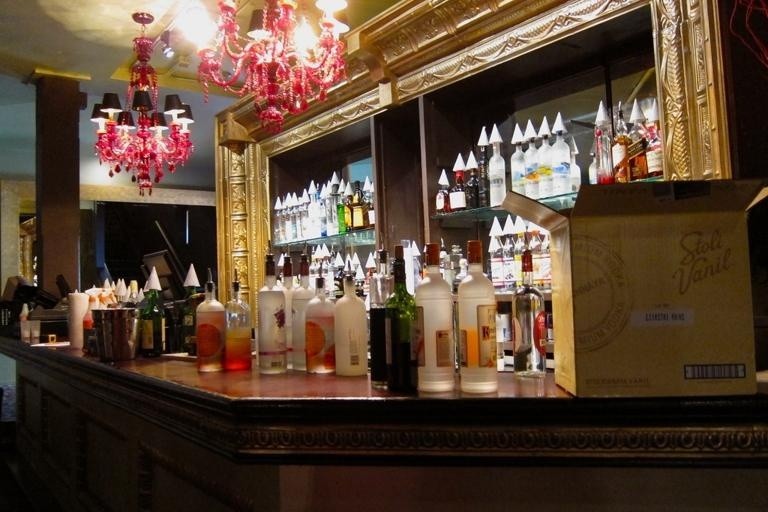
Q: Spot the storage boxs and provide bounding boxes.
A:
[501,178,768,399]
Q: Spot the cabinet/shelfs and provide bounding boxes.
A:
[213,0,768,399]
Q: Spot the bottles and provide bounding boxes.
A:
[20,304,43,339]
[68,212,556,395]
[273,171,376,243]
[435,98,663,217]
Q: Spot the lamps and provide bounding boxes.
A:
[89,12,193,196]
[197,0,350,134]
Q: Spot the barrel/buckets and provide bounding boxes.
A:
[92,309,138,360]
